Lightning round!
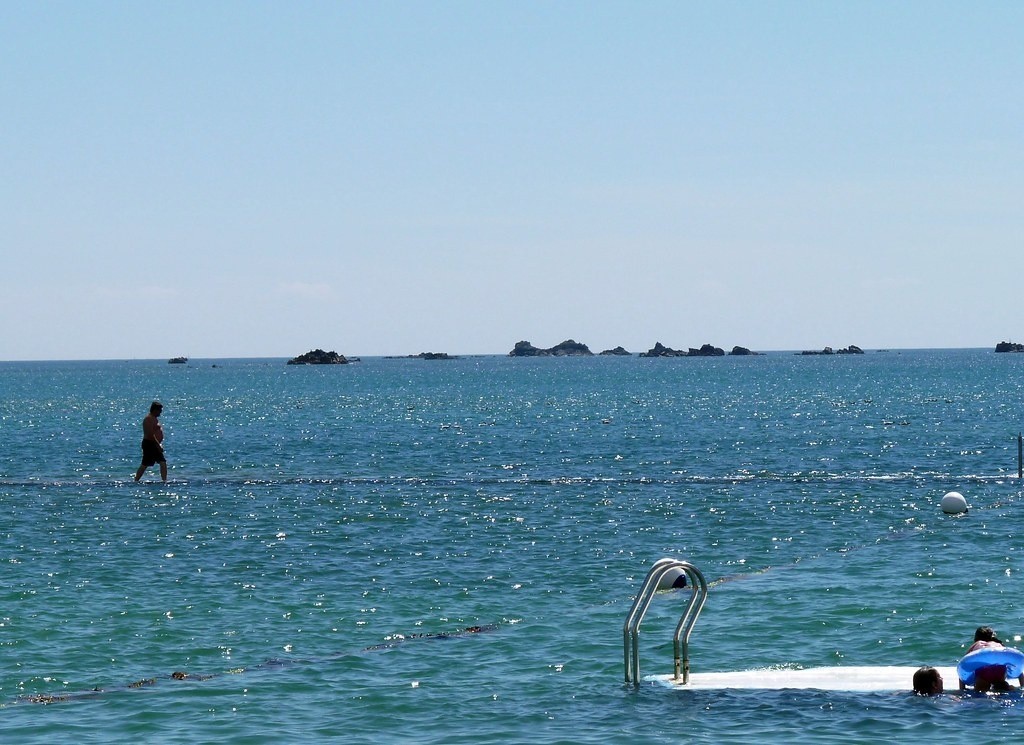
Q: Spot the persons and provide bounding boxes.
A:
[909,666,956,699]
[958,625,1024,696]
[134,401,168,482]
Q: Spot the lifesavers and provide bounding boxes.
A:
[956,646,1024,687]
